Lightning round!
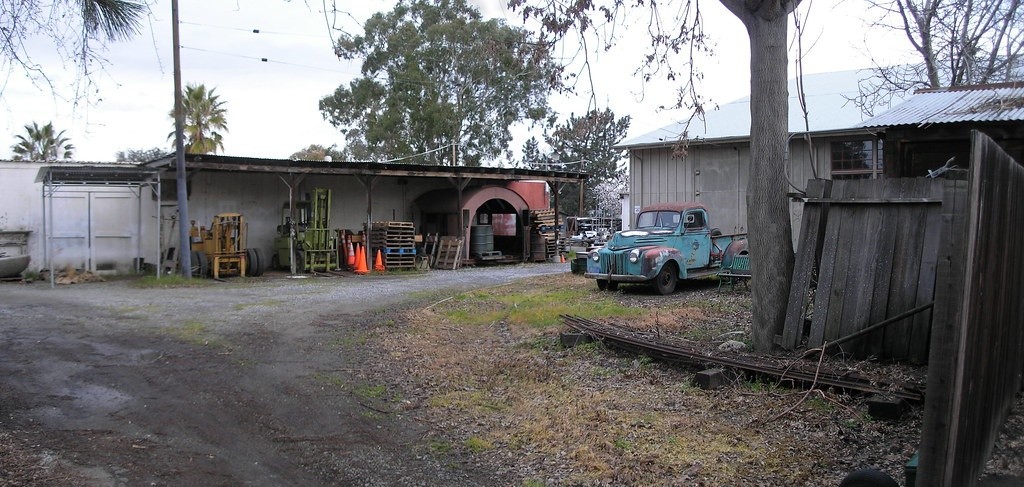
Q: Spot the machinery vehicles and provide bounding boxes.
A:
[189,211,265,279]
[271,184,340,273]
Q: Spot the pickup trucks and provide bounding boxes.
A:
[583,200,750,294]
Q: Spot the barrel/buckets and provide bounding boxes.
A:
[471,225,493,252]
[530,238,545,260]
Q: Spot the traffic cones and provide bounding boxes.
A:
[347,234,351,251]
[341,230,347,256]
[347,242,355,264]
[561,254,566,262]
[362,230,367,245]
[352,242,361,267]
[373,250,386,270]
[354,246,371,273]
